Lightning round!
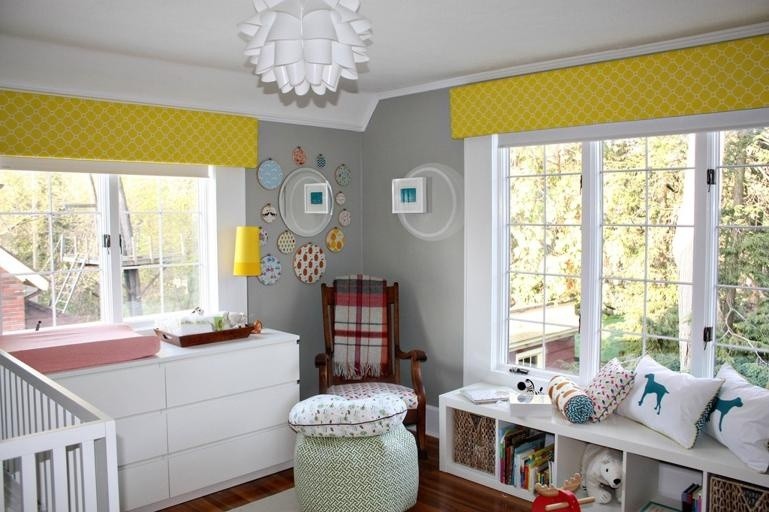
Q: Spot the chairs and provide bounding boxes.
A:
[315,273,428,460]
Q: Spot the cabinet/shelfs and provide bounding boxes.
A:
[48,328,301,512]
[439,384,769,512]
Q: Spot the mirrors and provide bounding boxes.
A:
[277,165,337,238]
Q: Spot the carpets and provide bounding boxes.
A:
[223,484,308,512]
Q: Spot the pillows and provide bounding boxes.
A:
[582,356,637,422]
[548,376,593,424]
[704,360,769,474]
[614,354,726,449]
[288,393,408,437]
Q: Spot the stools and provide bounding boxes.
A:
[293,430,419,512]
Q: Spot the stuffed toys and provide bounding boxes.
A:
[580,444,623,504]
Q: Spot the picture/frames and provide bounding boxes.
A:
[391,177,427,214]
[304,183,329,214]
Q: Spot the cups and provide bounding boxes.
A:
[213,313,242,331]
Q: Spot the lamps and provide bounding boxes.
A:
[237,0,374,96]
[233,226,261,333]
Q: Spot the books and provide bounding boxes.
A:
[500,427,554,492]
[681,484,703,512]
[461,387,514,404]
